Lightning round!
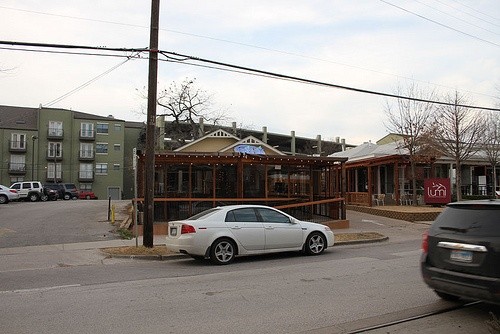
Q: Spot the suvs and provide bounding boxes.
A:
[40,184,65,202]
[57,183,78,200]
[419,200,500,312]
[9,181,43,202]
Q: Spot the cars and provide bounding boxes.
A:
[78,190,96,200]
[167,204,335,266]
[0,184,20,204]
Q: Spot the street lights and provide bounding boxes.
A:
[31,135,36,181]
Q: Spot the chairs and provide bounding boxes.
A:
[373,194,385,206]
[392,194,421,205]
